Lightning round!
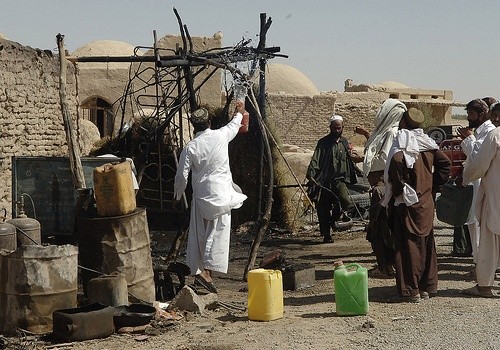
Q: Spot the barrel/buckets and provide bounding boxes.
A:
[93,161,136,215]
[333,261,369,316]
[247,268,283,321]
[3,244,79,335]
[80,207,156,306]
[87,272,128,307]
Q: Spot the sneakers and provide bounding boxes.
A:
[461,271,477,279]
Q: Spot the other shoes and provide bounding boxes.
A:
[391,294,420,303]
[323,236,333,242]
[446,252,467,257]
[330,220,339,229]
[420,292,429,299]
[467,253,473,257]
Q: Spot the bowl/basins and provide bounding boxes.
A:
[112,305,157,327]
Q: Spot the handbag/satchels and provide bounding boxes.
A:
[436,165,474,227]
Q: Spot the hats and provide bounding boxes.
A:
[330,115,343,122]
[191,108,208,124]
[464,99,489,113]
[404,107,424,128]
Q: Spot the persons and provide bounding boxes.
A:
[455,97,500,298]
[354,98,455,303]
[306,115,363,244]
[173,104,248,294]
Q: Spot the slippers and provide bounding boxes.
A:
[194,279,203,286]
[461,284,493,297]
[369,268,395,279]
[196,274,219,294]
